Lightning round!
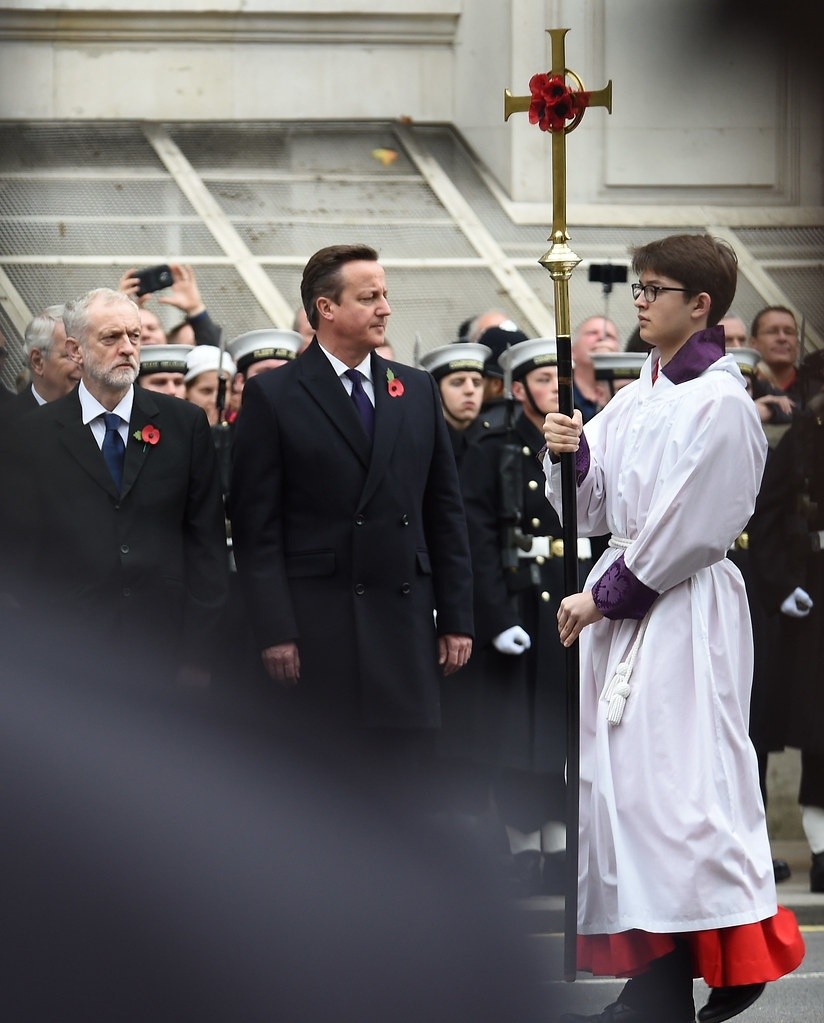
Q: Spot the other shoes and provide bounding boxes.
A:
[511,849,541,896]
[809,851,824,893]
[770,858,791,883]
[541,851,566,894]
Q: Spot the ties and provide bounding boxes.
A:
[97,412,127,495]
[344,369,375,443]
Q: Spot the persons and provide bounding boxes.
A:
[540,231,806,1023]
[0,244,823,899]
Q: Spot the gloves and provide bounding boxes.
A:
[780,586,813,618]
[490,625,531,655]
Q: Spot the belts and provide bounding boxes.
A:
[516,535,593,560]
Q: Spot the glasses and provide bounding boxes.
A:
[631,283,709,303]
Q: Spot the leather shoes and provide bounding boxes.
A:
[698,981,766,1023]
[560,1002,695,1023]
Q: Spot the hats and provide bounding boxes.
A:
[224,329,303,371]
[185,344,236,384]
[497,337,557,381]
[418,343,492,380]
[137,344,193,375]
[587,352,648,380]
[725,347,761,379]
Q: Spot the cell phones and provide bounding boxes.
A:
[588,263,628,285]
[127,264,174,298]
